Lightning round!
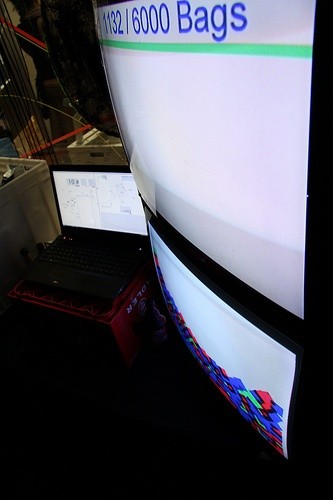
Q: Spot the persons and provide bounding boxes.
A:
[10,0,75,143]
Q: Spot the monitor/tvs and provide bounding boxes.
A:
[92,1,317,321]
[146,220,296,466]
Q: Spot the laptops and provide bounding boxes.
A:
[20,165,149,303]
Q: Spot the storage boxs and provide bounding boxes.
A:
[10,240,159,368]
